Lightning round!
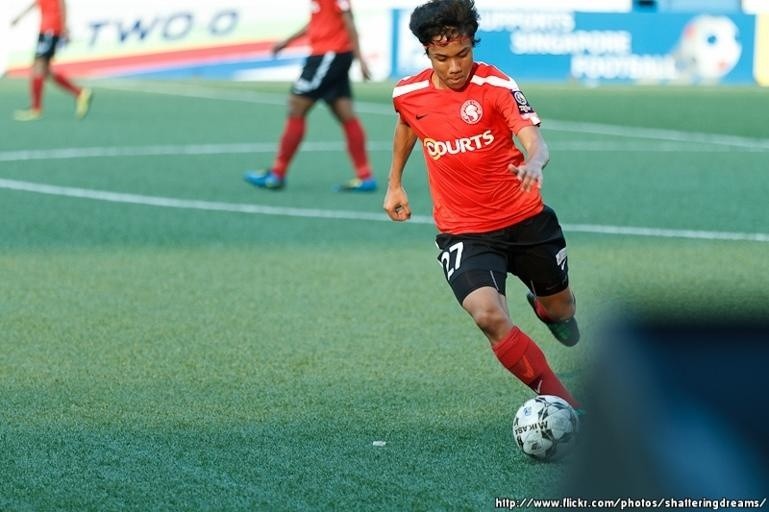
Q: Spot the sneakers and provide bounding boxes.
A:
[332,177,376,192]
[246,169,284,189]
[14,108,41,119]
[527,290,579,346]
[77,88,91,115]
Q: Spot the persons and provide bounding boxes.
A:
[6,0,93,123]
[240,0,378,193]
[382,0,587,424]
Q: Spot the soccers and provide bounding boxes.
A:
[512,396,578,464]
